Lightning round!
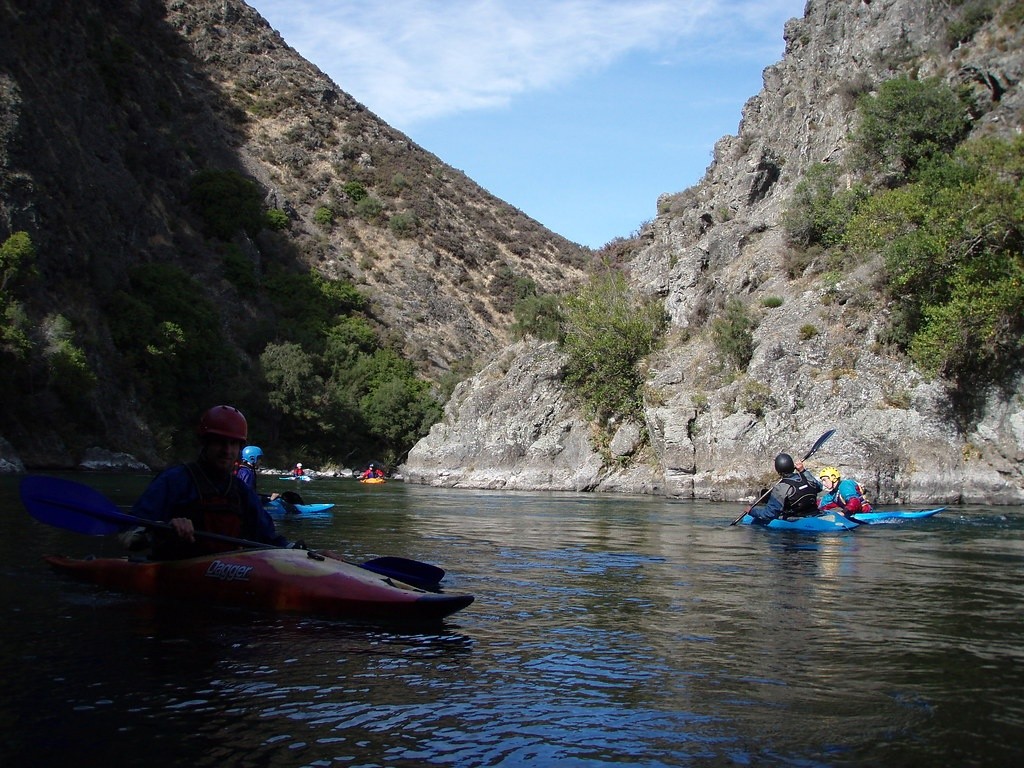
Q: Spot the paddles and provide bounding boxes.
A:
[730,429,836,525]
[257,490,306,507]
[19,475,446,588]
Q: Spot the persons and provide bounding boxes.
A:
[113,405,308,561]
[362,464,384,478]
[816,466,873,516]
[744,453,823,523]
[232,446,279,504]
[294,463,304,478]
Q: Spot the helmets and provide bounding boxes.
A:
[820,466,840,483]
[197,405,247,441]
[297,463,303,468]
[242,446,263,464]
[775,454,798,474]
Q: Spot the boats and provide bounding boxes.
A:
[259,491,336,515]
[278,476,313,481]
[360,478,386,483]
[851,506,947,524]
[740,513,860,532]
[35,547,475,619]
